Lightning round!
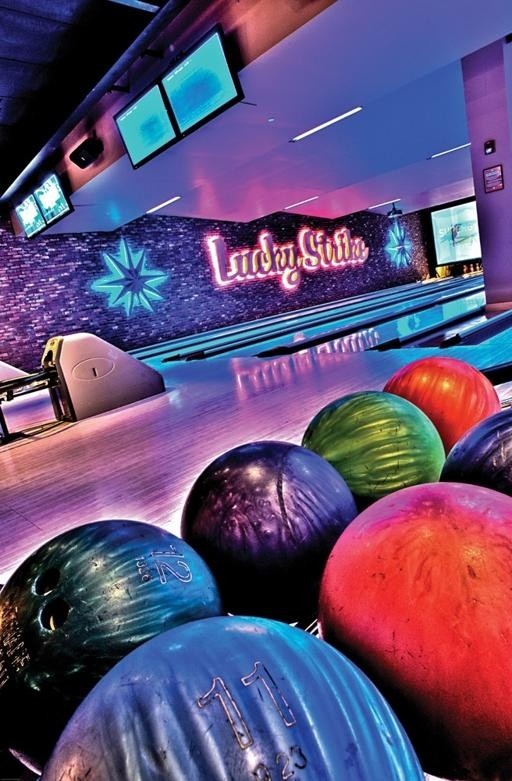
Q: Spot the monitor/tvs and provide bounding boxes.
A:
[32,170,75,225]
[14,193,48,240]
[113,81,181,171]
[157,22,246,139]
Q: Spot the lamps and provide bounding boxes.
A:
[387,202,403,219]
